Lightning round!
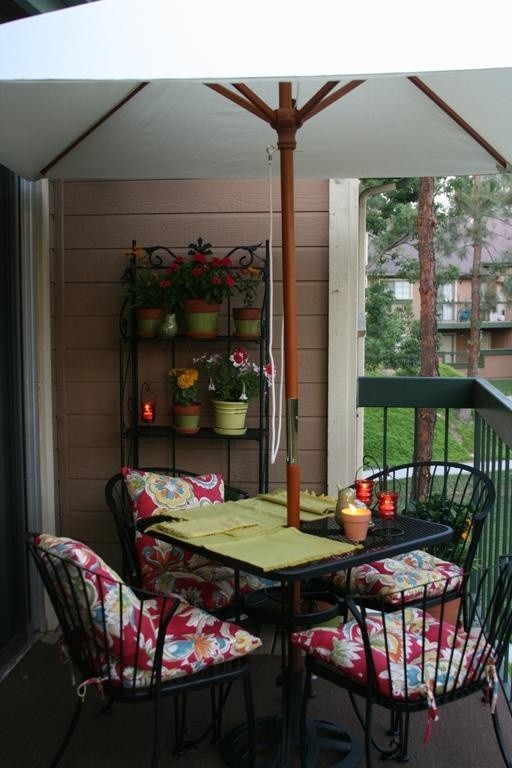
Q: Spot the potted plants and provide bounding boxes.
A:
[399,493,471,626]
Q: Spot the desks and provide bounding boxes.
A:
[136,489,454,768]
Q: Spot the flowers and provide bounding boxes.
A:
[232,267,263,307]
[166,367,199,406]
[169,252,235,303]
[191,345,277,400]
[159,279,180,313]
[120,243,159,308]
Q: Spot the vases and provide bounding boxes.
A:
[173,406,201,434]
[134,306,158,339]
[159,313,178,339]
[182,300,218,340]
[232,306,263,340]
[210,401,249,436]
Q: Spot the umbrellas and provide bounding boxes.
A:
[0,0,509,674]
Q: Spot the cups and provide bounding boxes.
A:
[340,509,371,541]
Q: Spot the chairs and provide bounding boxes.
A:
[340,461,495,633]
[288,552,511,768]
[104,466,285,654]
[20,527,263,767]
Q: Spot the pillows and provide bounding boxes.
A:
[121,466,247,593]
[28,532,158,672]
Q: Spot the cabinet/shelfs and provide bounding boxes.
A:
[117,238,272,501]
